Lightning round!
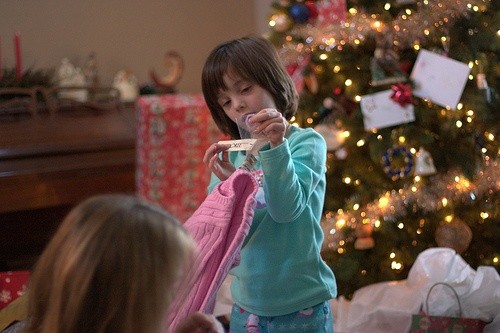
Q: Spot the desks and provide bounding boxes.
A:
[0,112,136,212]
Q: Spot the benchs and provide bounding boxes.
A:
[0,86,122,118]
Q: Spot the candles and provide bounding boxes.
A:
[13,32,22,77]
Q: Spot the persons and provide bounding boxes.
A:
[24,193,227,333]
[201,33,337,332]
